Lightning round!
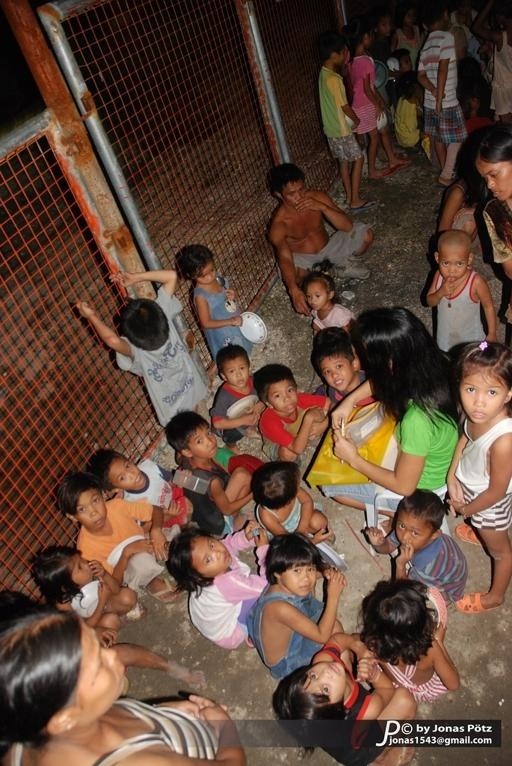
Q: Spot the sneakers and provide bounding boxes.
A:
[439,177,458,186]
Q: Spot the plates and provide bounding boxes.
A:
[225,395,259,419]
[309,535,346,570]
[373,60,388,88]
[388,56,401,70]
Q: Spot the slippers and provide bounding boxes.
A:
[344,159,413,211]
[125,602,146,622]
[455,524,480,545]
[455,592,500,613]
[148,576,187,603]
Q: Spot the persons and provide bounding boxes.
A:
[1,4,511,761]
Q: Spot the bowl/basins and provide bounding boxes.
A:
[239,312,267,344]
[172,470,210,495]
